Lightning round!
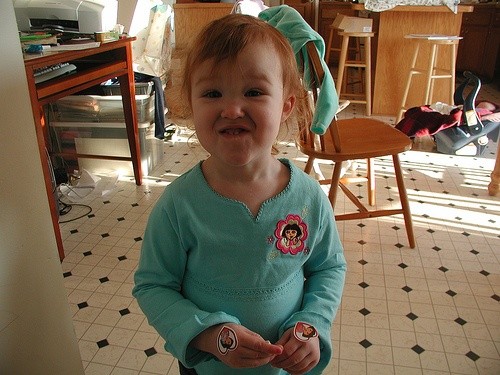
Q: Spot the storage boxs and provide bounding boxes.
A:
[50,74,169,178]
[331,13,373,34]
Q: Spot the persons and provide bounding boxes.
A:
[475,101,496,116]
[132,13,347,375]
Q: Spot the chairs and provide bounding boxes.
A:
[229,0,353,191]
[257,3,417,250]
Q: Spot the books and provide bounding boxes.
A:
[20,31,61,60]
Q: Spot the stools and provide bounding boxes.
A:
[335,31,374,115]
[394,37,460,125]
[325,25,363,98]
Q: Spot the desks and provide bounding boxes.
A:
[19,34,143,264]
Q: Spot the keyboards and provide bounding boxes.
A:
[33,63,77,83]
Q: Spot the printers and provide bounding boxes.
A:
[12,0,118,35]
